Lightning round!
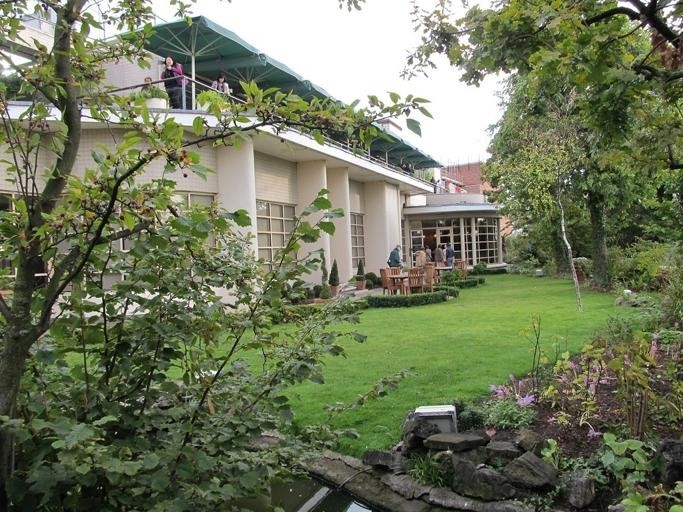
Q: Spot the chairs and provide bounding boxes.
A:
[425,259,465,279]
[379,266,434,295]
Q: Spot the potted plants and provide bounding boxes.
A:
[328,259,339,297]
[355,260,366,290]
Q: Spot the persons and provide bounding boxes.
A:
[401,162,407,170]
[406,164,414,174]
[349,134,356,145]
[209,71,231,96]
[159,55,187,109]
[387,244,403,270]
[139,76,157,94]
[436,179,440,185]
[430,176,435,184]
[413,241,455,269]
